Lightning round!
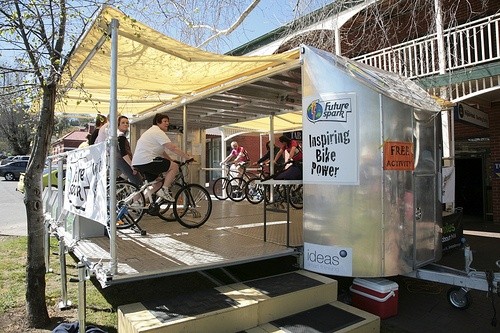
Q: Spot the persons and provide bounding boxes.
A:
[78,134,91,149]
[103,116,144,217]
[89,115,107,146]
[131,113,197,209]
[253,141,285,191]
[220,141,250,183]
[94,114,119,144]
[266,136,303,176]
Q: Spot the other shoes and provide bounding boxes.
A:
[132,210,147,215]
[233,190,241,193]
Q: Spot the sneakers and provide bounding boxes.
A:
[156,188,175,202]
[136,198,142,205]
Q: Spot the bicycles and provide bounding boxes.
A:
[213,159,304,209]
[106,157,213,230]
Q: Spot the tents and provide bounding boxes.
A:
[33,3,300,273]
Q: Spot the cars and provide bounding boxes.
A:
[0,155,49,180]
[16,165,67,194]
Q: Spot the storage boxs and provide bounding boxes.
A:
[349,278,398,318]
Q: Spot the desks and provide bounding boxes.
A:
[255,179,303,248]
